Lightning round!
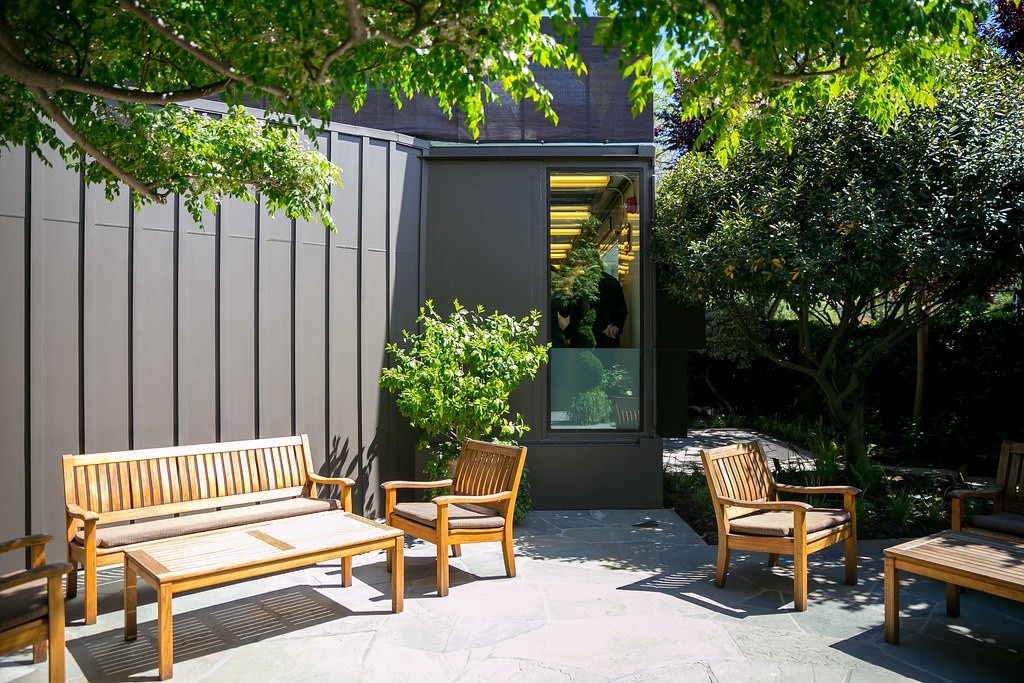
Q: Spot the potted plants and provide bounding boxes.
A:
[379,297,552,524]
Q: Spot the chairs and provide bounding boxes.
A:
[381,436,527,596]
[611,395,641,428]
[699,438,862,613]
[0,534,75,683]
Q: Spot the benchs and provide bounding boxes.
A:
[62,433,357,625]
[946,439,1024,620]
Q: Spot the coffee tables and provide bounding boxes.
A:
[121,509,405,682]
[883,528,1024,646]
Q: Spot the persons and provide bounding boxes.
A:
[590,270,627,423]
